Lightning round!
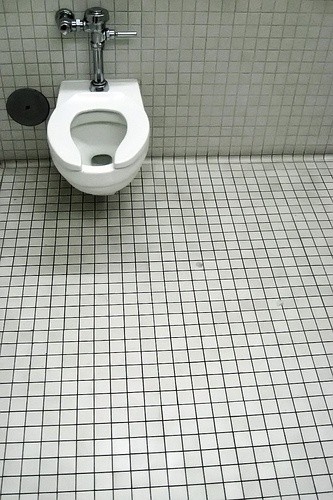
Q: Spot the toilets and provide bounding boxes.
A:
[47,79,150,197]
